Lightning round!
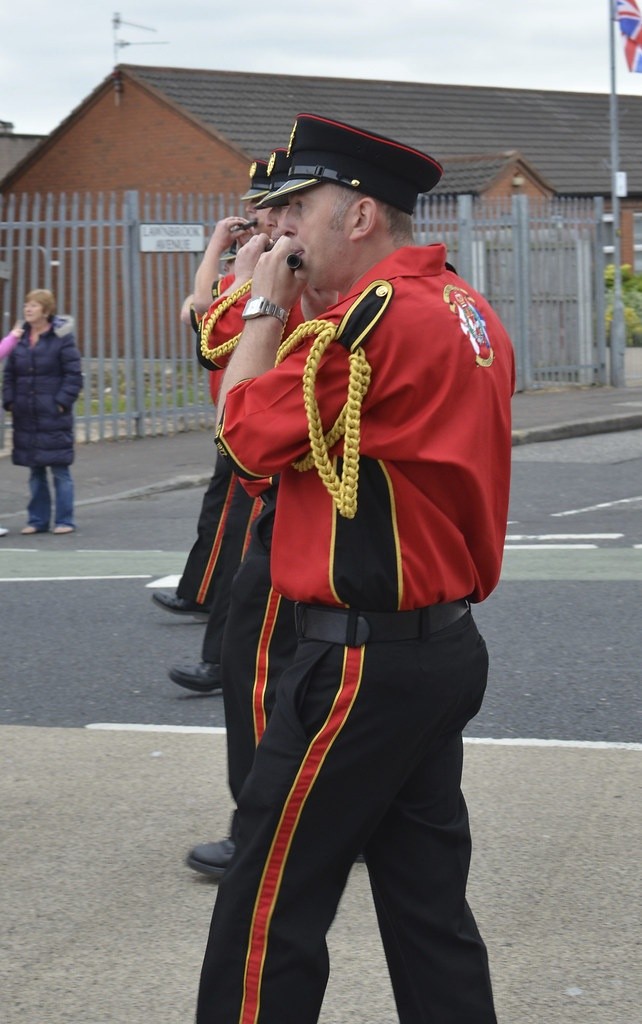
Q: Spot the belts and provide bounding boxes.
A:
[296,600,468,646]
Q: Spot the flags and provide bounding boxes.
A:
[612,0,642,73]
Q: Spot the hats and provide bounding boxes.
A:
[252,147,289,209]
[239,160,270,199]
[259,113,442,216]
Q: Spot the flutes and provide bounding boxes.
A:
[286,254,303,270]
[235,220,258,231]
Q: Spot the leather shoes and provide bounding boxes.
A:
[168,662,227,691]
[187,836,237,878]
[152,591,213,622]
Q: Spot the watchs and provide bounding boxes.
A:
[242,297,289,326]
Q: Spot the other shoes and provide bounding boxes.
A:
[0,528,9,536]
[20,524,41,534]
[52,524,74,534]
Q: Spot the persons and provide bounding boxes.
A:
[152,149,340,693]
[196,116,516,1024]
[0,289,83,537]
